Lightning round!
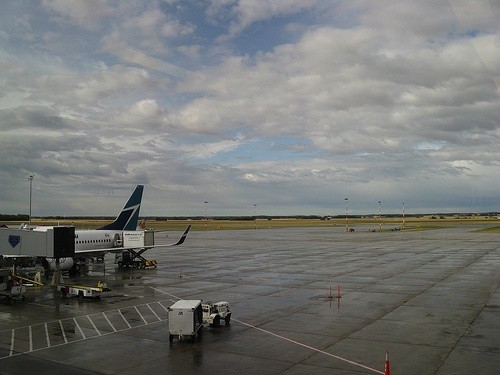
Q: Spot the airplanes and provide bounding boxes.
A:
[20,184,192,268]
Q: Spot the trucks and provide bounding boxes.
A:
[167,298,232,344]
